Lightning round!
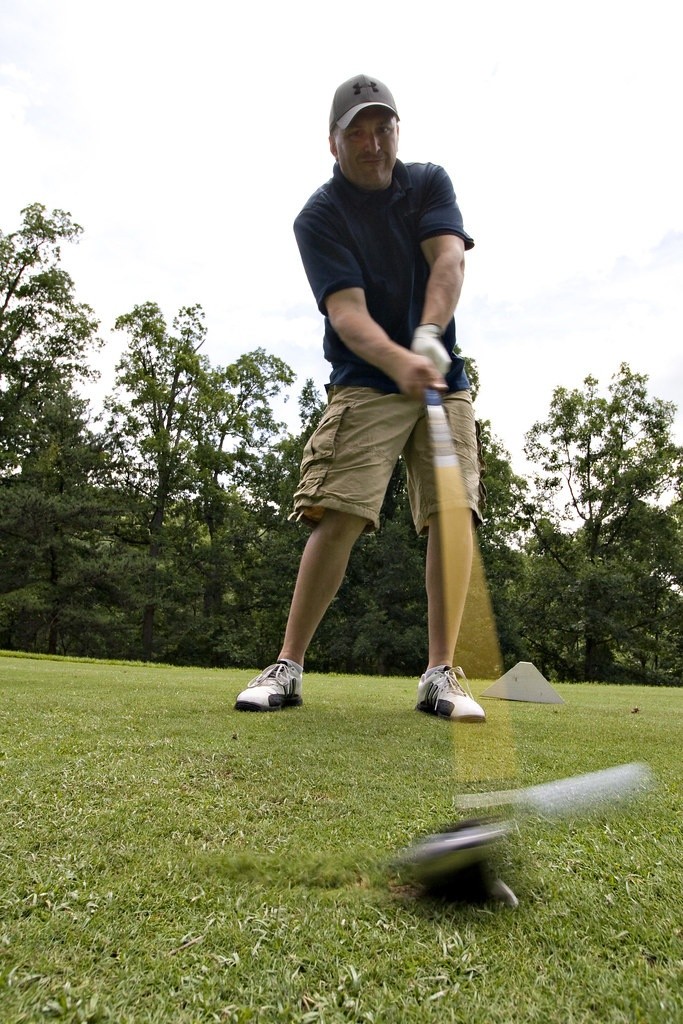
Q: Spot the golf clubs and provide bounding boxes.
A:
[402,386,523,880]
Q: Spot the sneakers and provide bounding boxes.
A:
[417,666,485,723]
[234,658,304,712]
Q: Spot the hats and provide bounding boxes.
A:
[328,75,400,133]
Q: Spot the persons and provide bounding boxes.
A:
[235,75,486,723]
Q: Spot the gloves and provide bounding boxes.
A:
[411,324,451,380]
